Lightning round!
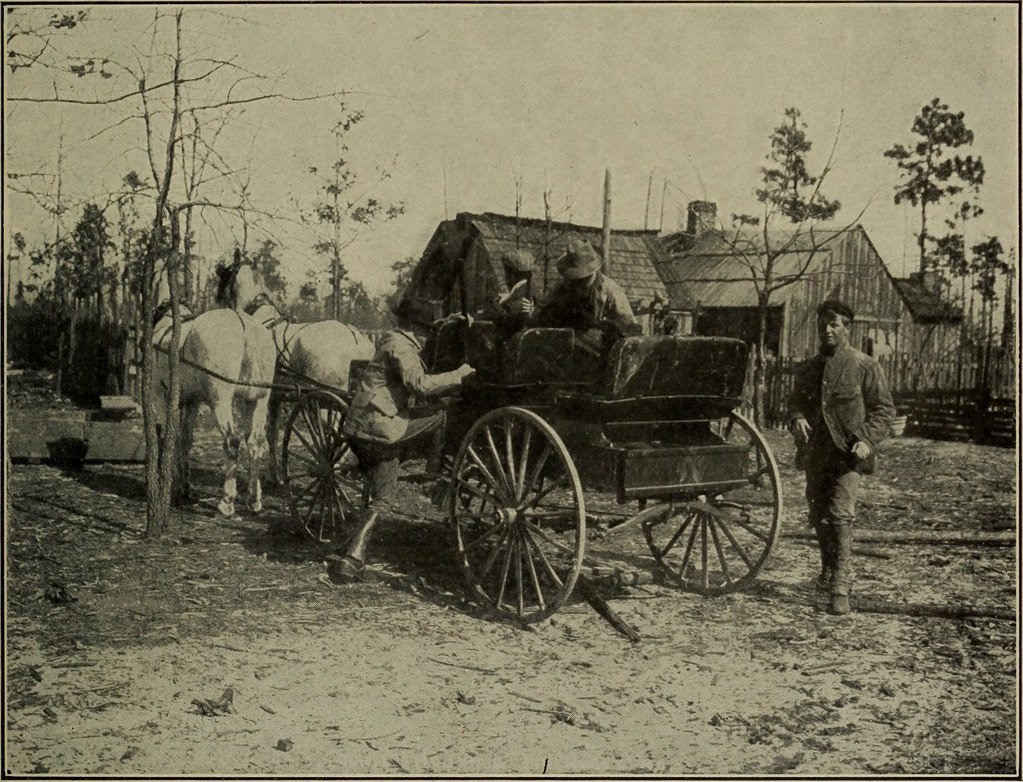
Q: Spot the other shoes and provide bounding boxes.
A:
[808,564,833,591]
[425,459,444,476]
[829,578,850,614]
[330,556,365,584]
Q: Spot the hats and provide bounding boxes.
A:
[391,297,435,328]
[556,238,605,280]
[502,250,534,272]
[817,300,855,321]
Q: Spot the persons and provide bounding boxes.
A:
[522,240,636,371]
[434,251,542,340]
[787,302,895,616]
[330,296,477,584]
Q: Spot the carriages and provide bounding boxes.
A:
[127,254,784,628]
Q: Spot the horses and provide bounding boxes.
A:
[132,246,373,521]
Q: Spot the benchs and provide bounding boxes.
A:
[552,334,751,424]
[474,326,609,403]
